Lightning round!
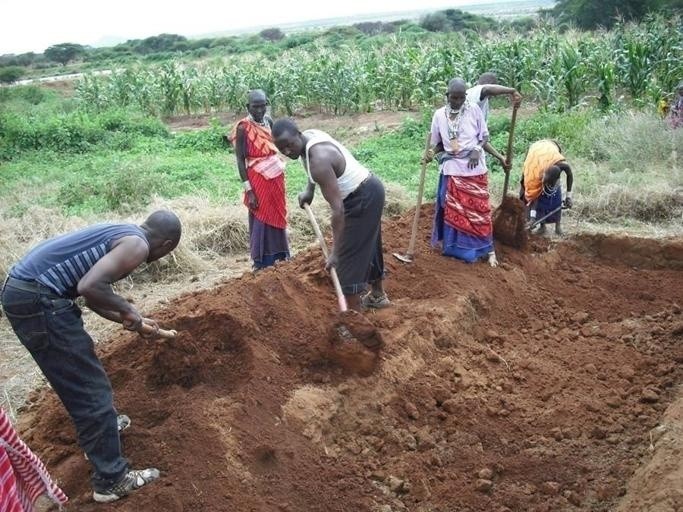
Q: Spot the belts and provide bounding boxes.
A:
[6,277,50,295]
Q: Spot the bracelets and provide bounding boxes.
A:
[428,148,435,157]
[566,192,572,198]
[529,210,536,218]
[473,145,483,152]
[241,180,252,193]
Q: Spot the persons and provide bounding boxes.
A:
[462,72,523,174]
[0,208,185,503]
[657,92,671,119]
[234,88,292,273]
[670,86,683,129]
[271,118,391,315]
[516,137,576,238]
[419,77,498,270]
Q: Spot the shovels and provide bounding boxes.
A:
[303,202,383,350]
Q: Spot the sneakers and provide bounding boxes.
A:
[93,468,160,503]
[84,415,131,460]
[359,291,390,307]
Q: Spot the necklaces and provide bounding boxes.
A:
[541,178,559,197]
